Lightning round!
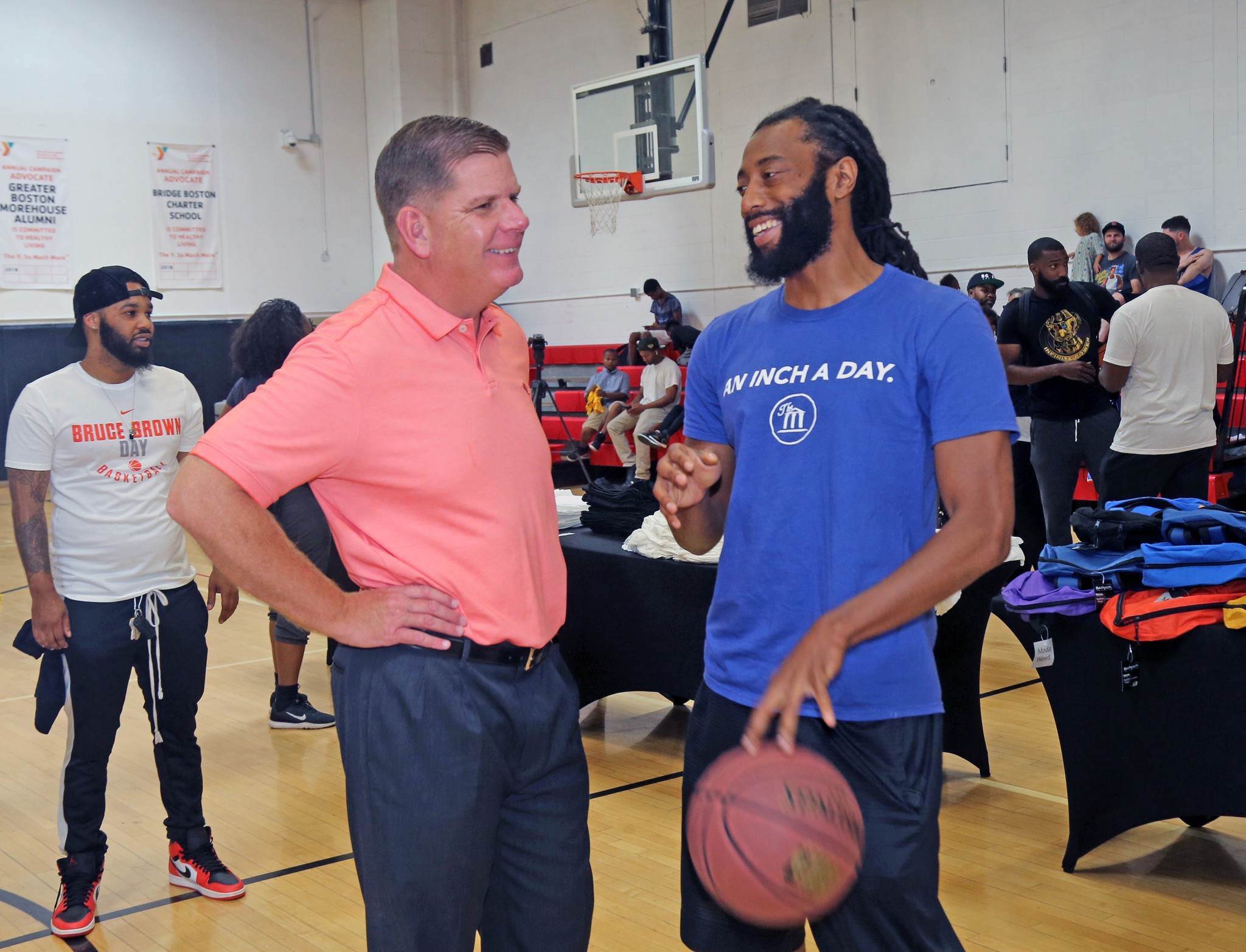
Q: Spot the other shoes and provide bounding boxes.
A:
[621,464,652,489]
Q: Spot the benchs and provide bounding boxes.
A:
[521,318,1246,515]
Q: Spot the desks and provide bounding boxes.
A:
[558,518,1020,779]
[989,593,1246,868]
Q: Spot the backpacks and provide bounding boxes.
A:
[1001,568,1097,623]
[1037,542,1246,595]
[1098,578,1246,642]
[1069,496,1246,554]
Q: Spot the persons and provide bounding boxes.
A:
[4,265,247,937]
[222,296,359,731]
[165,114,592,952]
[566,212,1235,545]
[656,98,1022,952]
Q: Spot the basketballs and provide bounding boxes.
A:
[689,740,865,927]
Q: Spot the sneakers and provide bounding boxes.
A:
[648,429,671,448]
[168,826,246,901]
[566,445,589,462]
[637,430,661,448]
[589,431,606,452]
[50,854,106,938]
[268,682,336,729]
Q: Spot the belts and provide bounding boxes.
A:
[439,638,551,670]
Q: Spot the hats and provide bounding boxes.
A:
[967,272,1005,291]
[637,336,660,351]
[65,266,163,347]
[1102,222,1125,234]
